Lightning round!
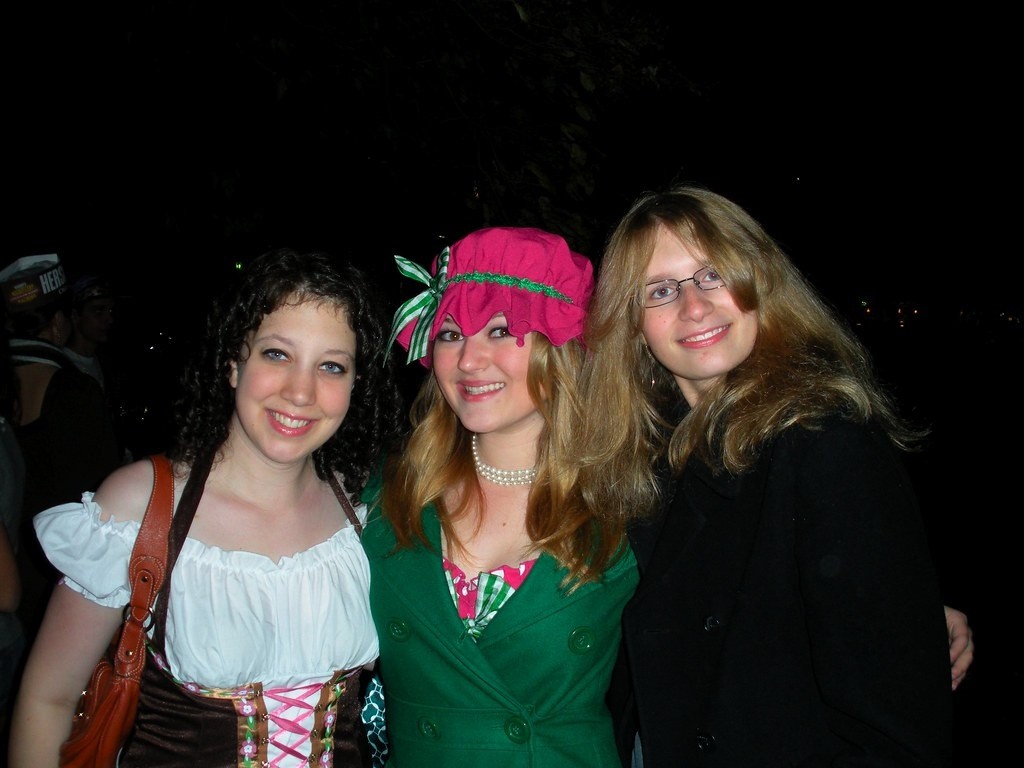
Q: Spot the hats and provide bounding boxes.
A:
[383,226,596,368]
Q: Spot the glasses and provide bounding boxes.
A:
[631,263,726,310]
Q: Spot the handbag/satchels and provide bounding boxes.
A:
[59,453,175,768]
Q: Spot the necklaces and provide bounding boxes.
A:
[471,432,535,485]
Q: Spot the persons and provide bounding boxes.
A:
[570,190,961,768]
[7,251,393,768]
[0,251,125,741]
[360,226,973,768]
